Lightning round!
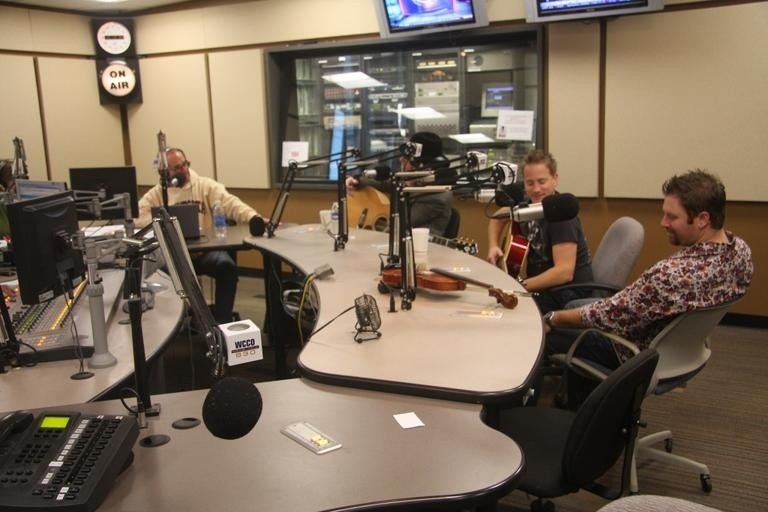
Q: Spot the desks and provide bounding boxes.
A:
[237,215,550,404]
[0,263,186,411]
[131,218,297,253]
[27,373,530,512]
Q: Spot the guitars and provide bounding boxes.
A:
[346,186,479,255]
[495,221,531,286]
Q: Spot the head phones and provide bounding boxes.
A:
[0,136,29,190]
[165,148,191,182]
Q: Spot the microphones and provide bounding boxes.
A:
[202,375,262,440]
[415,168,458,185]
[492,194,579,221]
[463,185,524,206]
[248,215,266,236]
[170,173,186,187]
[363,166,391,181]
[405,148,423,168]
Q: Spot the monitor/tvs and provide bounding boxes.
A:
[68,166,139,221]
[151,205,200,238]
[526,0,664,23]
[16,180,67,200]
[373,0,489,39]
[6,191,85,307]
[481,82,514,117]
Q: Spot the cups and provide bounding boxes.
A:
[414,253,429,271]
[320,209,333,227]
[410,228,430,252]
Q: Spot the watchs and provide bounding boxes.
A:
[543,311,555,329]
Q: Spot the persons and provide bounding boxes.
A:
[527,169,753,410]
[130,148,264,322]
[487,149,595,310]
[344,131,452,237]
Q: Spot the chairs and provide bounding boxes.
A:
[443,207,461,241]
[545,290,744,500]
[481,327,660,511]
[540,213,649,303]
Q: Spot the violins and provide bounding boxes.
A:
[382,263,518,309]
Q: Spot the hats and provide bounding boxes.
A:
[400,132,450,170]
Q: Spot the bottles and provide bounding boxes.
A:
[329,202,340,234]
[486,149,496,171]
[212,201,229,241]
